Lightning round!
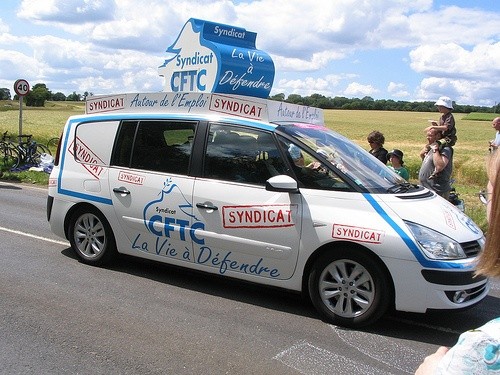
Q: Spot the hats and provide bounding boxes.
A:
[434,96,454,111]
[386,149,404,163]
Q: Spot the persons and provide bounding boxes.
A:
[386,149,409,181]
[489,116,500,152]
[367,131,388,165]
[288,143,313,178]
[410,145,500,374]
[420,97,457,180]
[419,127,454,197]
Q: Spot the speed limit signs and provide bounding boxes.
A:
[15,79,30,96]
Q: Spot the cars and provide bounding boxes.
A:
[47,16,490,331]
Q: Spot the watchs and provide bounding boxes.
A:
[432,149,439,153]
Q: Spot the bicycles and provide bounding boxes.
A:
[0,130,60,174]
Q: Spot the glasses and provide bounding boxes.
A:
[479,191,493,204]
[368,141,376,144]
